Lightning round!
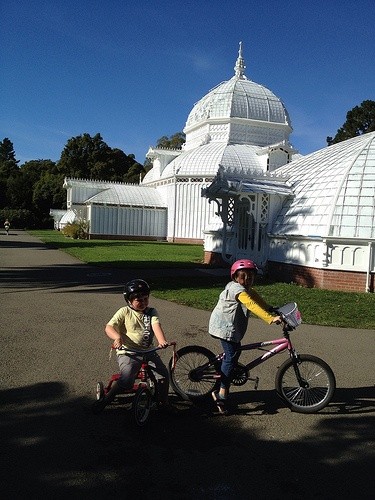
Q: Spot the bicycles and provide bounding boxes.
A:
[168,302,336,413]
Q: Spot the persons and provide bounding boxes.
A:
[89,279,182,419]
[4,219,11,237]
[208,258,282,415]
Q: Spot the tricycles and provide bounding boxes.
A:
[96,344,163,425]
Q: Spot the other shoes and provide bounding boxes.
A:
[156,400,179,415]
[93,395,111,414]
[212,389,228,414]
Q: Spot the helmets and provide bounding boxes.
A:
[231,259,258,280]
[123,278,150,302]
